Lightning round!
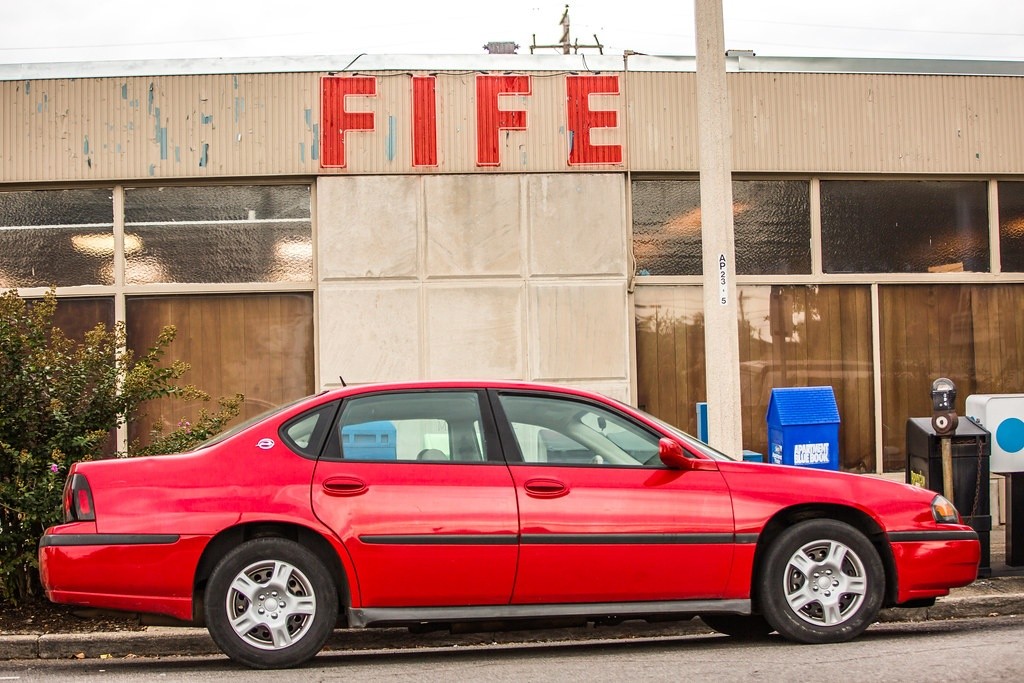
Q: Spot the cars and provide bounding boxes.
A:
[39,381,982,670]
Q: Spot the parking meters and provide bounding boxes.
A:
[928,378,959,508]
